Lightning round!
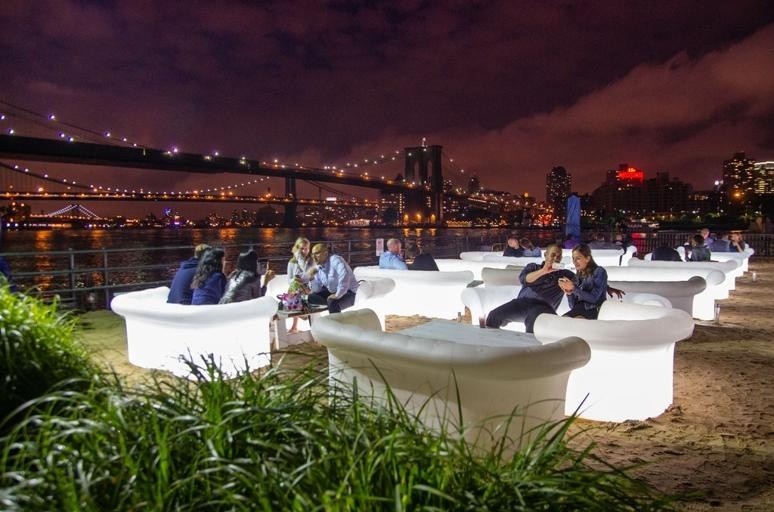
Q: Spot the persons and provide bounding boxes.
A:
[503,235,525,258]
[519,236,543,258]
[286,237,313,333]
[190,247,227,306]
[218,249,275,306]
[651,215,773,262]
[486,242,627,333]
[377,236,408,271]
[555,243,608,320]
[166,243,212,304]
[306,243,360,313]
